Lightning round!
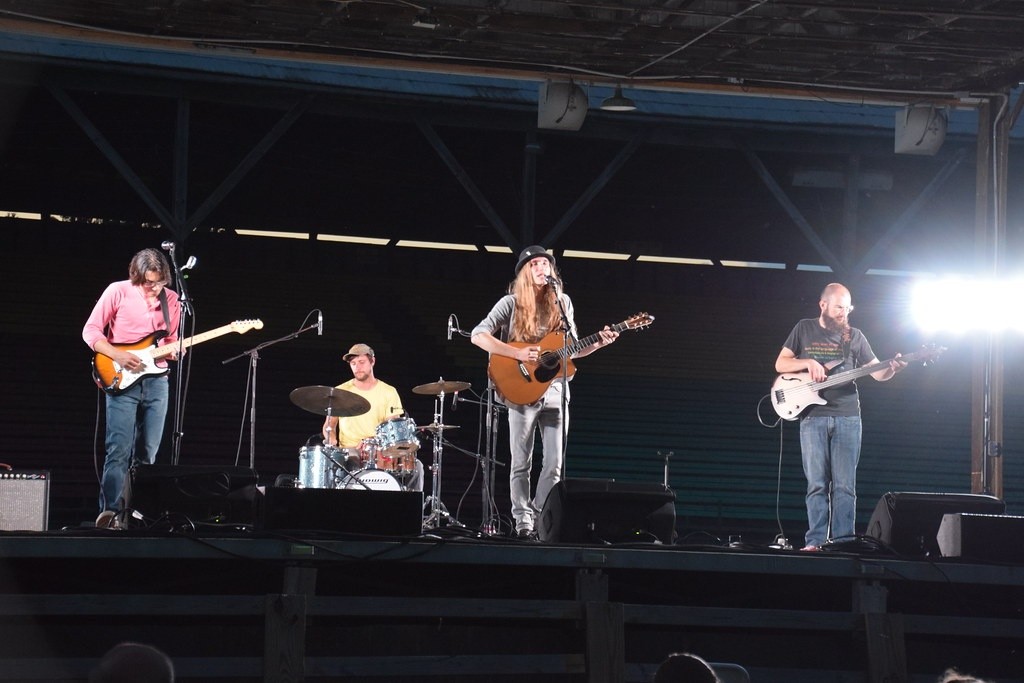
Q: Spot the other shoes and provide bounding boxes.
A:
[799,544,822,550]
[95,510,119,530]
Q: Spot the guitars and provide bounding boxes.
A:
[90,318,264,398]
[770,345,949,422]
[487,311,656,412]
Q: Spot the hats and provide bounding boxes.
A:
[515,245,556,280]
[343,344,374,364]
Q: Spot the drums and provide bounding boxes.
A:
[361,438,415,470]
[298,445,365,489]
[376,414,422,458]
[334,465,406,492]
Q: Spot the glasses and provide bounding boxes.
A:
[831,304,854,314]
[142,280,168,288]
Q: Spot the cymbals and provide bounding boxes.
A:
[411,381,472,394]
[289,384,372,417]
[414,424,461,431]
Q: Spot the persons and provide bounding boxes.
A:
[654,654,720,683]
[88,639,174,683]
[80,247,187,528]
[471,245,620,538]
[774,282,908,552]
[322,344,425,496]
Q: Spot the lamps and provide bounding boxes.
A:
[891,97,948,158]
[600,76,638,112]
[536,79,589,132]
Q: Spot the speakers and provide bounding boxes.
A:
[0,469,50,532]
[935,513,1024,564]
[538,478,676,546]
[115,464,259,533]
[865,491,1006,554]
[248,484,424,537]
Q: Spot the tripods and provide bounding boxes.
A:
[419,390,470,533]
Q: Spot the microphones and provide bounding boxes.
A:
[451,391,459,409]
[318,312,323,335]
[448,315,453,340]
[161,240,176,250]
[545,277,559,285]
